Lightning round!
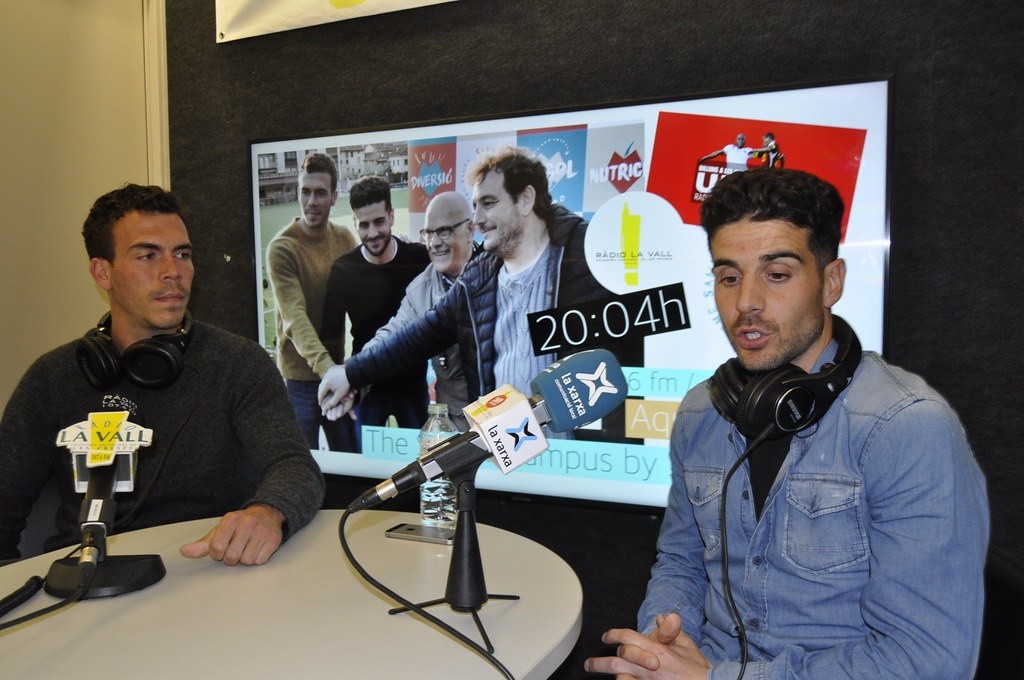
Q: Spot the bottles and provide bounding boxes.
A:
[420,403,459,525]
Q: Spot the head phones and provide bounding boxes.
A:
[77,305,192,389]
[707,312,862,440]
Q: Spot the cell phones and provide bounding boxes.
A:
[385,523,457,545]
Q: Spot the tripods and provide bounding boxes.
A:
[389,466,521,654]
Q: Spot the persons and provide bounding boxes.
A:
[584,168,990,680]
[263,152,606,452]
[699,132,785,169]
[0,185,326,566]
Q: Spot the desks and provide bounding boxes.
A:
[0,510,584,680]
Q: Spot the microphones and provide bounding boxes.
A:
[346,347,628,514]
[56,388,154,589]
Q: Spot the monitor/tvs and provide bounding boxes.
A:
[245,75,895,523]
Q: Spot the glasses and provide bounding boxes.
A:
[420,218,470,241]
